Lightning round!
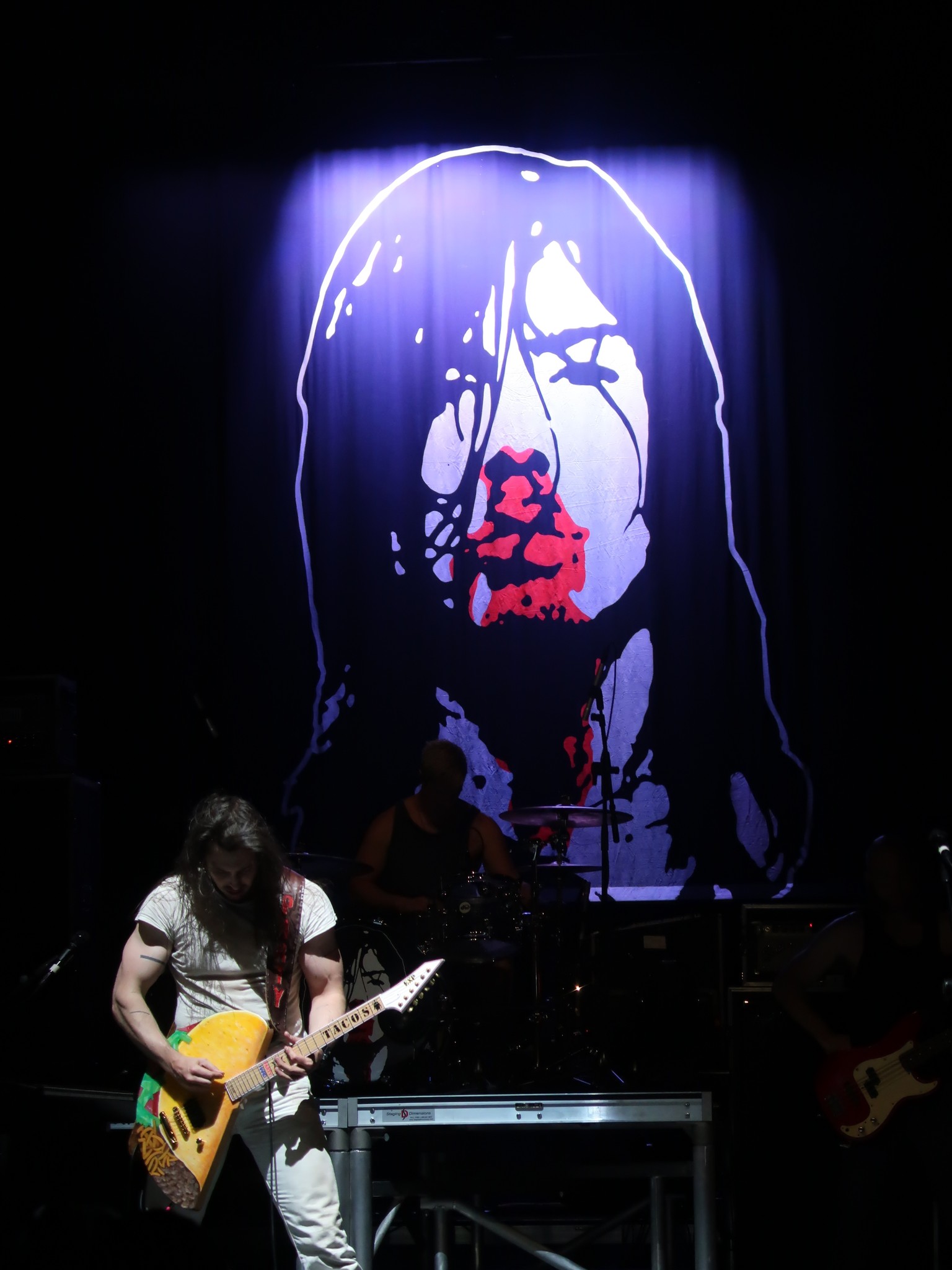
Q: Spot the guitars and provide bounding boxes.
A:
[136,958,445,1212]
[813,1009,952,1140]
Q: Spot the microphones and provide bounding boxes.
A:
[583,649,608,720]
[39,929,89,984]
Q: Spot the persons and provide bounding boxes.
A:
[113,794,363,1270]
[774,831,952,1270]
[349,742,532,972]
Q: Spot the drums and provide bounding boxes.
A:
[440,873,523,967]
[302,919,441,1086]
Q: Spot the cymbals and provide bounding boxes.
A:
[285,851,375,873]
[499,807,633,828]
[520,860,602,873]
